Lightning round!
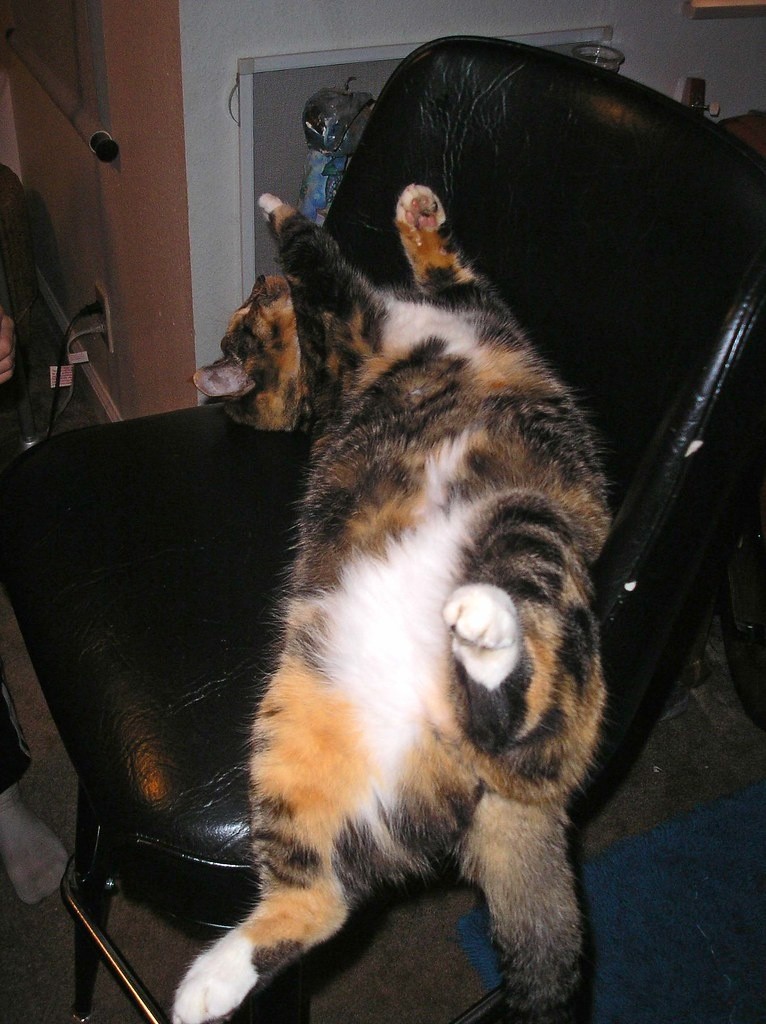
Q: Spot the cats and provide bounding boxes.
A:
[171,182,613,1024]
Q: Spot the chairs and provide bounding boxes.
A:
[0,35,766,1024]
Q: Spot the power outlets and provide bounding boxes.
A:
[95,281,114,353]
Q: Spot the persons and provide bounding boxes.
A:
[0,304,69,904]
